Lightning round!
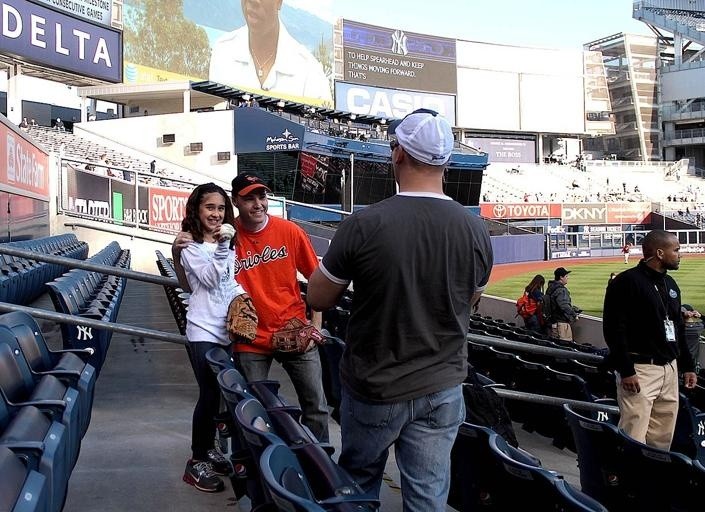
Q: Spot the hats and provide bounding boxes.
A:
[680,304,693,320]
[554,266,571,280]
[231,171,272,197]
[388,108,455,166]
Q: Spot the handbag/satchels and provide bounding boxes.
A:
[517,294,538,319]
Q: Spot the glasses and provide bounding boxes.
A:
[389,139,399,151]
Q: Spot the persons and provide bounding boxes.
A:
[676,206,705,227]
[607,272,615,285]
[622,243,629,264]
[545,266,579,342]
[19,116,29,128]
[29,118,39,126]
[54,117,65,131]
[681,303,705,363]
[522,274,545,330]
[171,170,330,446]
[603,228,698,455]
[482,153,700,203]
[306,113,495,507]
[180,181,245,495]
[81,154,169,189]
[207,0,332,109]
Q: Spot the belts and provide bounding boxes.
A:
[630,352,667,368]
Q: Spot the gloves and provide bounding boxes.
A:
[227,295,259,344]
[270,317,326,356]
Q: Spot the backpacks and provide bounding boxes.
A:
[515,294,536,319]
[542,283,561,314]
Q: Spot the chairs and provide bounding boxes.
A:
[451,392,705,512]
[155,251,196,336]
[300,280,355,426]
[465,313,704,450]
[0,230,129,512]
[206,346,382,506]
[16,123,232,197]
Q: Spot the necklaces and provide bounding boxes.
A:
[249,44,275,77]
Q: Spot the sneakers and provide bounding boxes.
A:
[183,459,223,493]
[207,450,234,475]
